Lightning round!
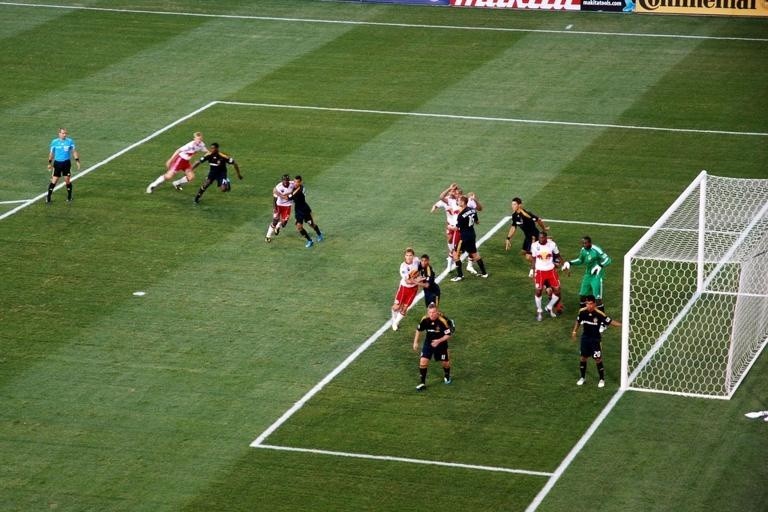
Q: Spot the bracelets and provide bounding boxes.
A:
[75,158,79,161]
[48,160,52,163]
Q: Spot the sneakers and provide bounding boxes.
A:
[392,323,399,331]
[265,234,271,243]
[172,181,183,191]
[576,377,586,386]
[146,185,154,194]
[305,238,313,248]
[597,378,605,388]
[316,232,323,243]
[416,383,426,391]
[536,309,544,322]
[544,304,557,318]
[392,321,400,327]
[466,266,478,275]
[476,273,489,278]
[450,275,464,282]
[444,375,451,385]
[274,222,280,235]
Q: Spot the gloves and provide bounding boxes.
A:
[528,269,534,278]
[590,264,603,276]
[561,261,571,272]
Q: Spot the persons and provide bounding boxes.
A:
[273,175,324,248]
[264,174,305,243]
[146,132,209,194]
[191,142,244,207]
[45,127,82,204]
